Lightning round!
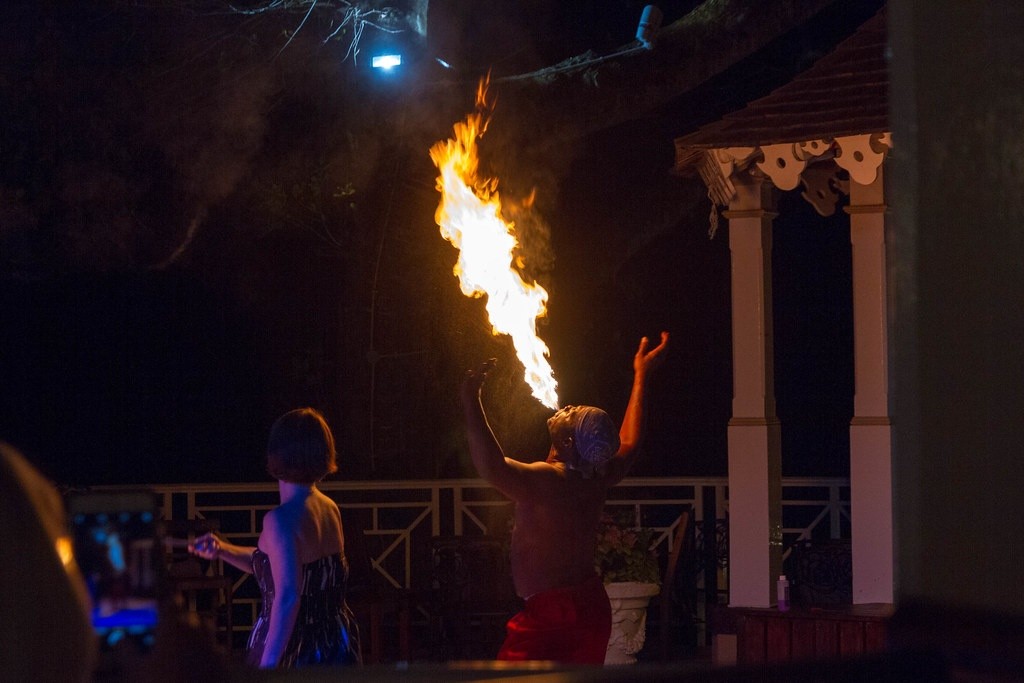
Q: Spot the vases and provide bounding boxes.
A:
[602,582,660,666]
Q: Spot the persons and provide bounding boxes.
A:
[187,407,362,670]
[0,441,98,683]
[459,331,668,660]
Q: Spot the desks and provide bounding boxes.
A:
[736,603,901,665]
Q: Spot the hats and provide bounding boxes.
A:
[575,407,622,465]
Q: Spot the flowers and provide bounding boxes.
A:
[594,526,662,586]
